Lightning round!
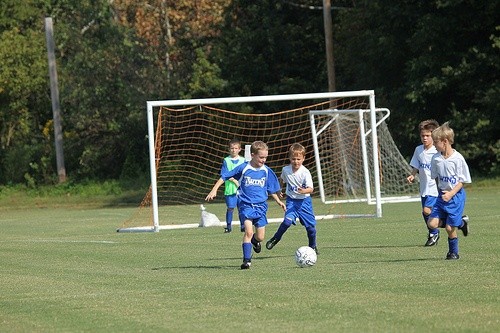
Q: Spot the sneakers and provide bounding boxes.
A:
[425,232,439,247]
[460,215,469,237]
[240,259,251,269]
[241,227,245,232]
[225,228,230,233]
[444,252,459,260]
[251,234,261,253]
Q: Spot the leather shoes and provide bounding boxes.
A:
[266,237,278,249]
[313,246,319,254]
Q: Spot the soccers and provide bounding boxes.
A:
[295,246,318,268]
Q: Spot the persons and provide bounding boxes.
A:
[204,141,287,270]
[220,142,250,234]
[405,120,442,247]
[426,121,473,261]
[265,143,320,255]
